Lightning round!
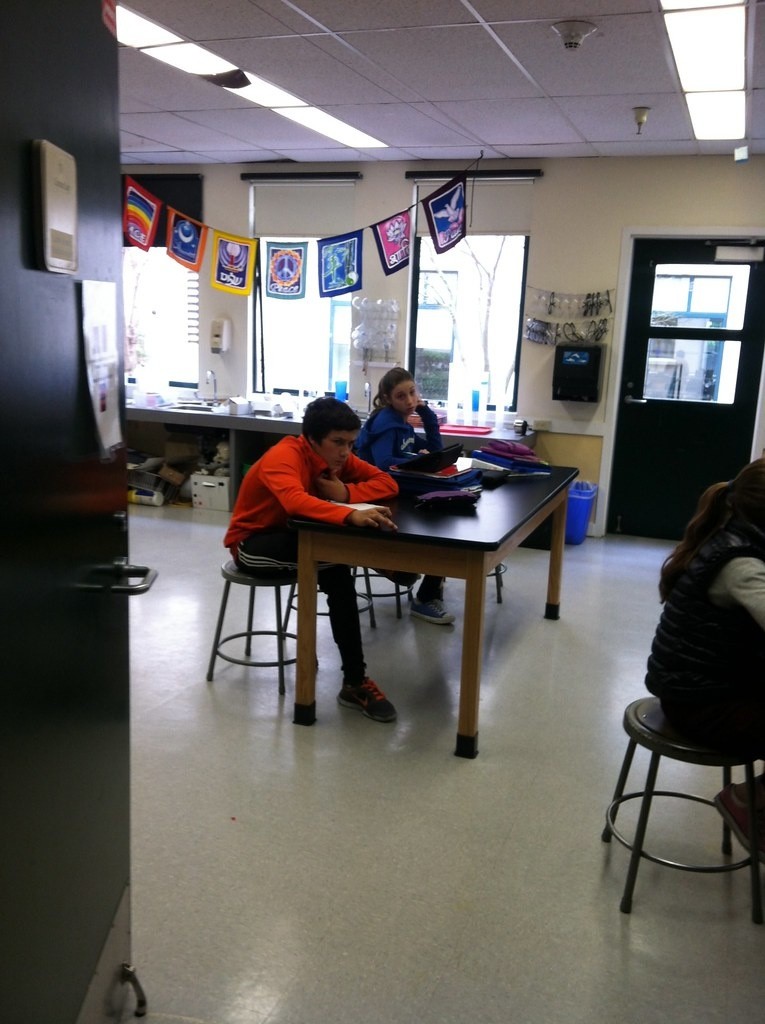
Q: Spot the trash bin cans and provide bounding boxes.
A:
[566,481,598,544]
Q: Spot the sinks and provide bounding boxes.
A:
[168,406,213,412]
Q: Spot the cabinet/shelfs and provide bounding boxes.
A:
[125,405,536,513]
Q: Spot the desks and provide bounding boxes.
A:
[286,465,580,757]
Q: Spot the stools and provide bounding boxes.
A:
[599,699,765,926]
[206,554,509,696]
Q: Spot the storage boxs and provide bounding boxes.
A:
[229,396,253,415]
[126,423,230,512]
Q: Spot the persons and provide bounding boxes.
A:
[355,367,456,625]
[223,396,419,721]
[645,459,764,862]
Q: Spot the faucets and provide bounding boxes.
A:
[205,369,218,402]
[363,381,371,420]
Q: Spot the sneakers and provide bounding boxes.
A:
[713,781,765,864]
[410,598,455,623]
[337,680,396,720]
[372,568,418,586]
